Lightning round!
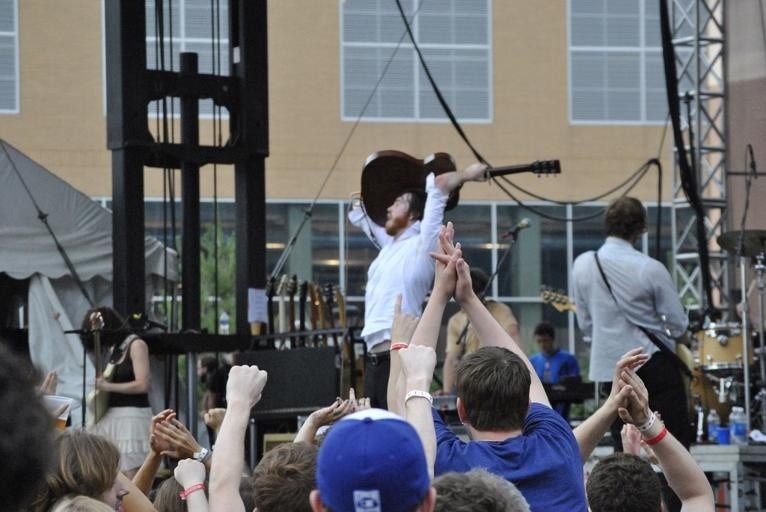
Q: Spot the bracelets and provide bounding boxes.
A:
[197,449,208,461]
[180,484,205,499]
[637,413,656,431]
[645,428,667,445]
[405,391,432,403]
[391,342,408,350]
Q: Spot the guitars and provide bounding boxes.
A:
[266,275,347,349]
[540,292,693,386]
[360,150,558,226]
[88,313,112,428]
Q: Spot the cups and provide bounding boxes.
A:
[717,425,729,446]
[43,393,74,431]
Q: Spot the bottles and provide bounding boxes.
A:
[730,405,748,446]
[706,409,721,442]
[218,312,230,336]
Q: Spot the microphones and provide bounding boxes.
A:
[507,215,534,236]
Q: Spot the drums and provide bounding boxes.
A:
[699,327,753,375]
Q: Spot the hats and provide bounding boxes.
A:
[314,407,430,512]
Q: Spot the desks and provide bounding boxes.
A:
[586,445,766,512]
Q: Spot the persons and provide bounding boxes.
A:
[349,163,489,411]
[80,308,154,482]
[395,222,589,512]
[0,293,437,512]
[435,268,519,395]
[530,323,582,418]
[621,411,682,473]
[572,347,715,512]
[573,199,692,512]
[433,469,530,512]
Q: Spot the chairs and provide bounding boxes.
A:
[233,346,335,470]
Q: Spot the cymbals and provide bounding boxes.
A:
[717,230,766,257]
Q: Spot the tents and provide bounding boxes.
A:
[0,139,177,429]
